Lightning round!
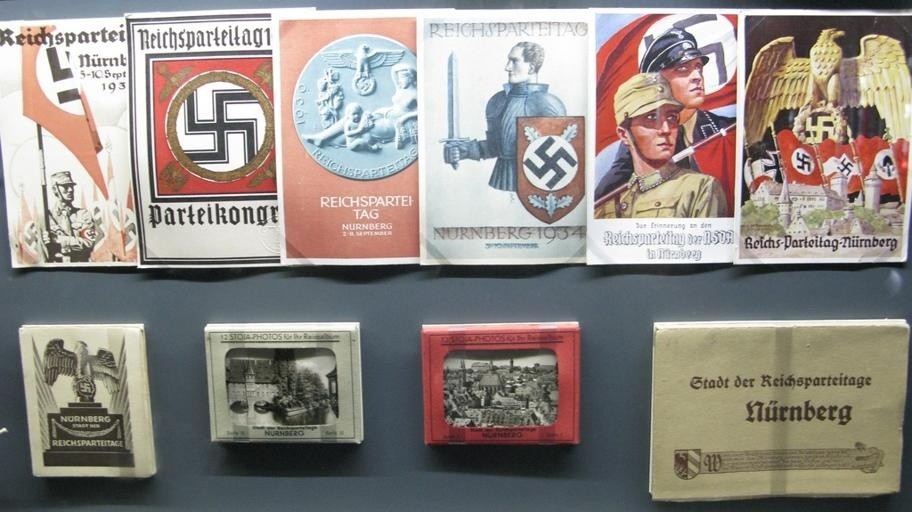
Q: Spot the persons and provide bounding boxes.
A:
[594,27,736,197]
[444,41,570,193]
[594,70,728,218]
[344,102,384,153]
[36,171,97,262]
[304,63,418,153]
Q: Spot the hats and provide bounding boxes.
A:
[641,27,708,72]
[614,73,682,127]
[48,171,75,189]
